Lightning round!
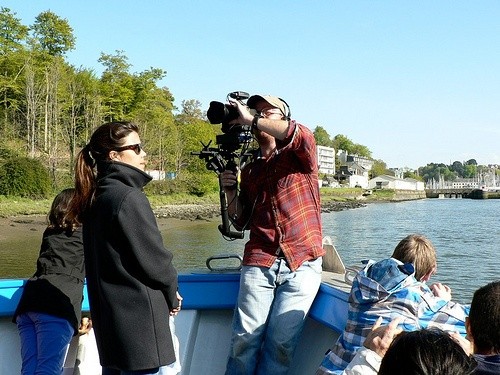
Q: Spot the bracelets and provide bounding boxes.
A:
[252,114,264,130]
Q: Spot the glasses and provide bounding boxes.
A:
[255,109,282,117]
[107,143,142,158]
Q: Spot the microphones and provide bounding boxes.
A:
[278,98,291,120]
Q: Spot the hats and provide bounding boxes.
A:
[247,94,286,117]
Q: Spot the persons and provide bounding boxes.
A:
[466,281,500,375]
[76,120,183,375]
[221,95,323,375]
[317,235,471,375]
[12,189,84,375]
[341,317,472,375]
